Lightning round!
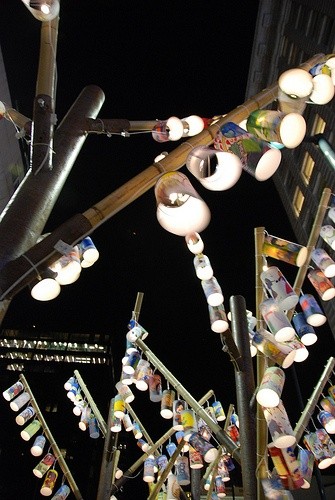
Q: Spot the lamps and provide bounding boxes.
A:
[22,0,61,20]
[2,54,335,500]
